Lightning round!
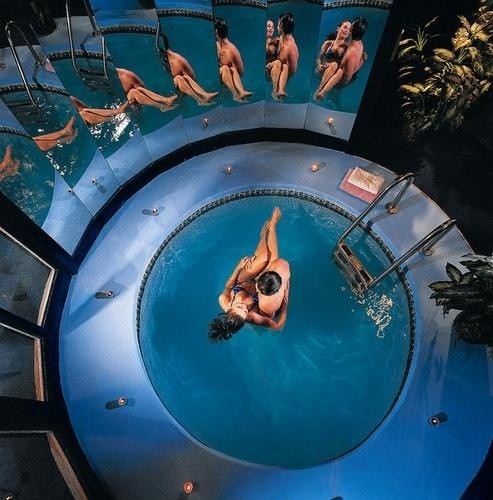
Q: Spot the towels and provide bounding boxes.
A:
[348,166,385,196]
[338,169,383,204]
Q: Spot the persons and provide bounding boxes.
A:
[115,67,178,114]
[162,33,217,106]
[69,93,129,126]
[32,115,77,153]
[313,15,368,104]
[266,11,299,102]
[208,205,291,345]
[1,144,21,184]
[213,18,252,103]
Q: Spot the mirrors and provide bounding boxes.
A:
[2,1,119,218]
[154,1,224,141]
[13,0,152,185]
[2,99,94,258]
[305,0,394,141]
[265,0,323,130]
[89,0,189,161]
[209,0,266,135]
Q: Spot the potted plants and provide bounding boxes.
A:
[428,253,493,344]
